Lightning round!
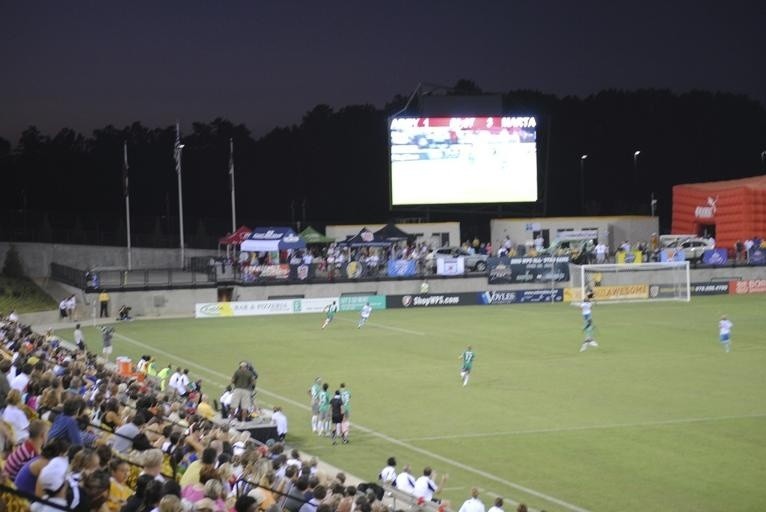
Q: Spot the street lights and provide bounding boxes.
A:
[632,147,641,173]
[580,154,588,207]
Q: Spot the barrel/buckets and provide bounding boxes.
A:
[87,271,100,289]
[116,356,132,375]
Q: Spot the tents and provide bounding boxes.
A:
[220,223,413,261]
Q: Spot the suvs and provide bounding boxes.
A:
[424,246,490,273]
[538,235,598,264]
[652,237,714,264]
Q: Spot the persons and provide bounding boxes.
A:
[708,234,715,250]
[229,241,435,277]
[717,314,734,354]
[614,239,644,265]
[456,345,478,388]
[100,289,109,318]
[651,233,657,250]
[463,235,608,264]
[579,320,599,352]
[571,298,593,330]
[60,294,76,320]
[734,236,766,264]
[584,286,594,302]
[0,310,258,512]
[319,300,338,329]
[357,302,373,329]
[119,305,127,319]
[256,377,530,511]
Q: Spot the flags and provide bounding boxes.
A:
[227,153,235,193]
[121,163,131,199]
[172,131,182,174]
